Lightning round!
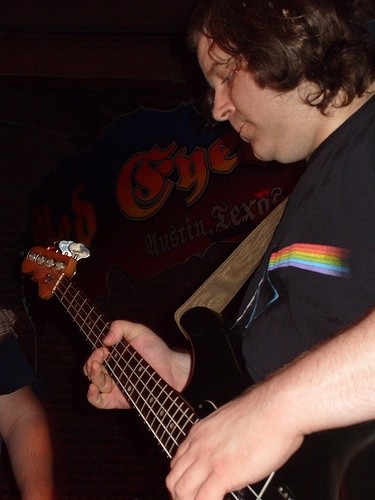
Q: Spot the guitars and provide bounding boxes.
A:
[20,238,300,500]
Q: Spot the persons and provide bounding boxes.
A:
[83,0,375,500]
[1,331,57,500]
